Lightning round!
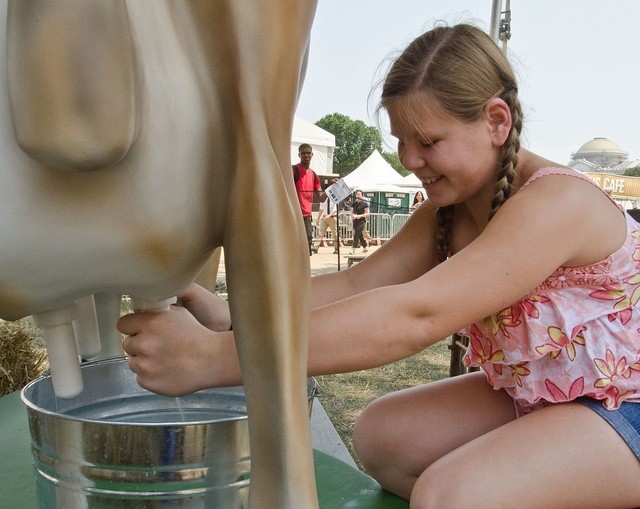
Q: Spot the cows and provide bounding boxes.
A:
[0,0,320,509]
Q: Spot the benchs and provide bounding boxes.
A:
[343,255,365,267]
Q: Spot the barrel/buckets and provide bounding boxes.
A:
[20,356,325,508]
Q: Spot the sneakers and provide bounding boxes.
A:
[333,249,338,254]
[311,248,318,253]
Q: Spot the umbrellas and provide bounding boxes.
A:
[339,147,414,236]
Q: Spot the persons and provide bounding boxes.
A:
[310,183,340,254]
[118,24,640,509]
[343,197,352,210]
[292,143,340,256]
[350,190,369,255]
[411,191,425,212]
